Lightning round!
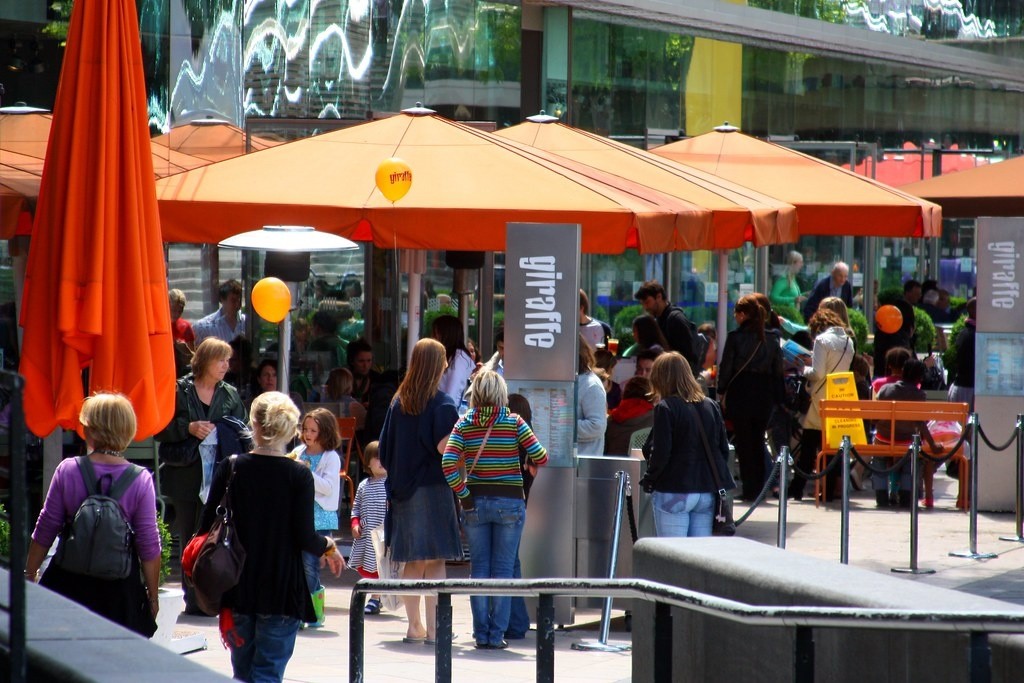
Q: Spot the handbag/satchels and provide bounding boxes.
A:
[192,515,245,615]
[922,359,945,390]
[794,383,813,414]
[713,495,735,537]
[158,380,199,464]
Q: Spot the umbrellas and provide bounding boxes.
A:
[18,0,176,443]
[647,121,941,391]
[157,98,702,380]
[897,153,1024,217]
[0,103,289,237]
[484,110,796,253]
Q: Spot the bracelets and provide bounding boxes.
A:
[323,540,336,557]
[24,567,37,576]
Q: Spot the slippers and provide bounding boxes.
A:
[403,630,427,644]
[424,631,457,644]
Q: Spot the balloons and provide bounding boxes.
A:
[252,277,292,325]
[875,305,902,333]
[376,158,412,204]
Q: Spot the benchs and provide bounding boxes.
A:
[817,398,968,510]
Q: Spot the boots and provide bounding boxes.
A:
[773,479,805,501]
[892,488,912,510]
[298,585,324,627]
[876,487,891,509]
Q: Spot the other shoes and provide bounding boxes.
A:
[477,639,509,650]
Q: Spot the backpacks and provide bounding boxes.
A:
[664,308,708,373]
[53,455,146,579]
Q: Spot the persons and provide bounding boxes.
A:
[25,384,161,639]
[802,260,977,513]
[154,273,389,683]
[577,252,871,537]
[377,313,606,648]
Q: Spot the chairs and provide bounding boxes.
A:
[336,416,355,509]
[628,427,654,515]
[124,433,169,523]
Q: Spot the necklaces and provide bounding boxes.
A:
[93,448,123,457]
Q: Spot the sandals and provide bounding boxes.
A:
[366,599,383,615]
